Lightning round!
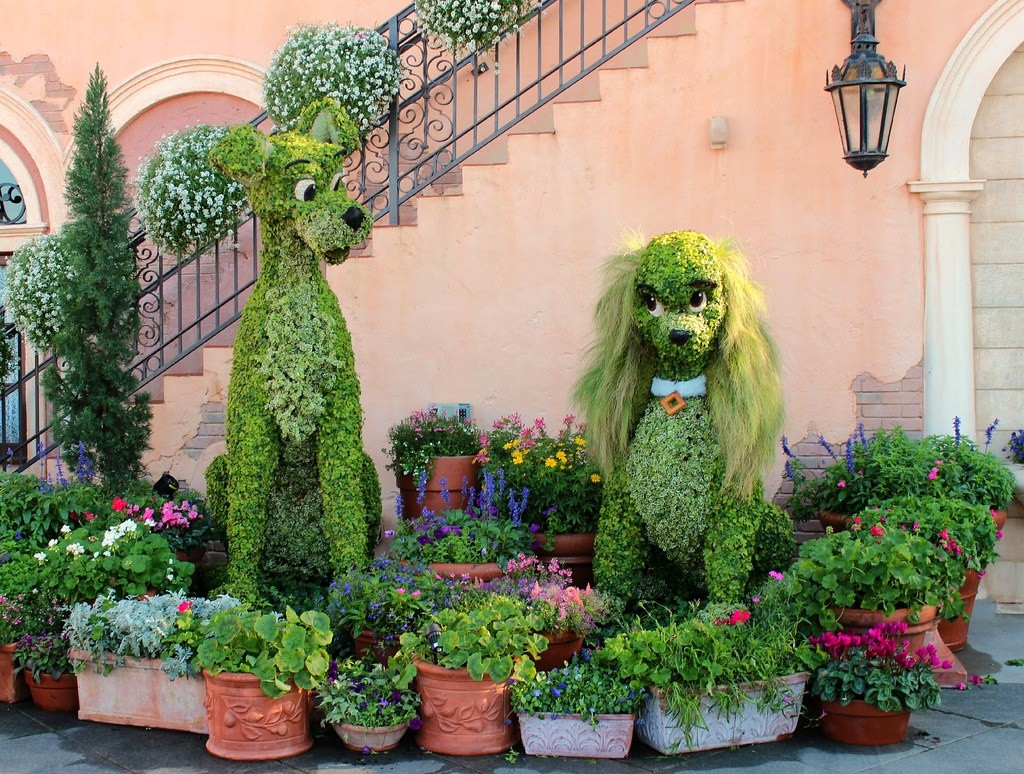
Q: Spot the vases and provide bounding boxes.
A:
[0,455,1005,761]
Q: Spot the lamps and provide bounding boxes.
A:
[822,1,906,178]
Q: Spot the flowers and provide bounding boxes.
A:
[777,426,912,516]
[790,519,967,620]
[0,0,829,727]
[852,488,1000,579]
[1001,429,1024,463]
[903,415,1016,508]
